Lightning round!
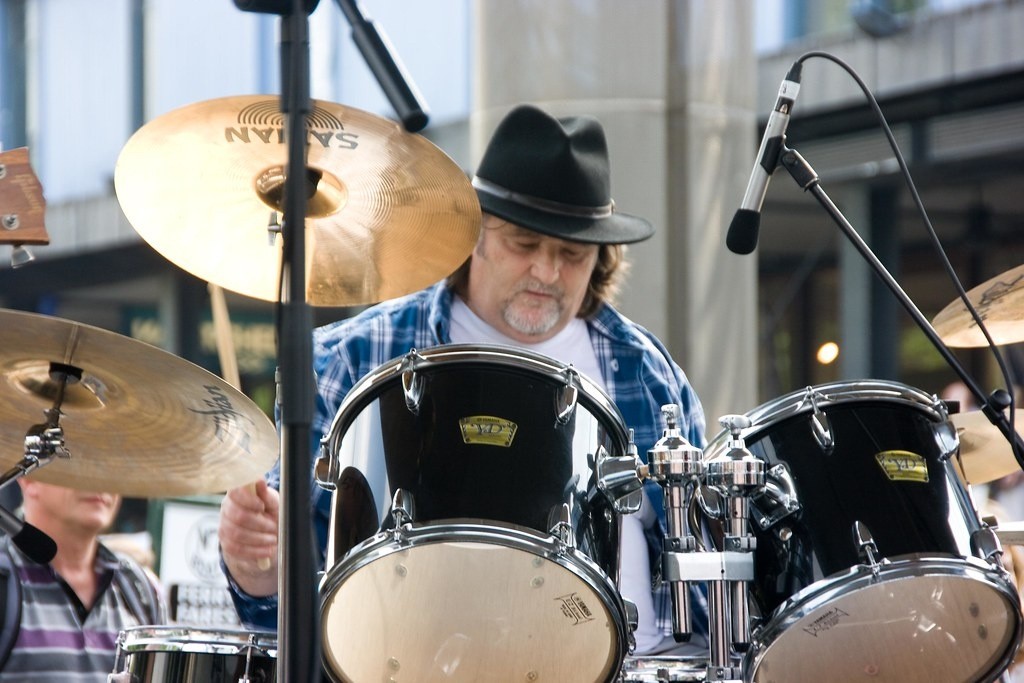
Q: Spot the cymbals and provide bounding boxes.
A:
[947,408,1024,485]
[0,308,280,499]
[931,262,1024,349]
[113,95,482,307]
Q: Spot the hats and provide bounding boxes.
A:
[470,104,654,248]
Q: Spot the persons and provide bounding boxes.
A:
[0,473,174,683]
[218,130,723,661]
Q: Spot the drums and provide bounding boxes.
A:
[702,378,1023,683]
[316,342,643,682]
[108,624,277,683]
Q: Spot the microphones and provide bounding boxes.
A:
[725,61,803,255]
